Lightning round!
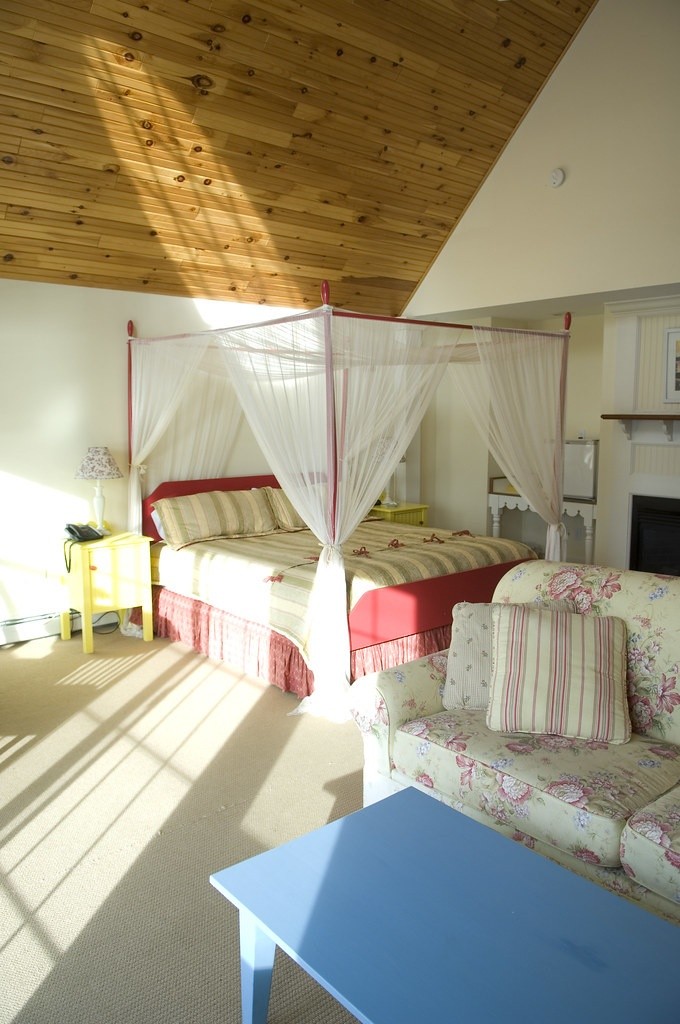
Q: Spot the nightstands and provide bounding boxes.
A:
[369,503,430,527]
[60,527,154,653]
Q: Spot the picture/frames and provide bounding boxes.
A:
[663,328,680,404]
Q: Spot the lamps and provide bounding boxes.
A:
[74,447,124,535]
[373,440,406,505]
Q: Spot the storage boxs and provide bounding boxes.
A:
[544,436,600,499]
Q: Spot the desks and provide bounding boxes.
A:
[210,786,680,1024]
[488,476,597,564]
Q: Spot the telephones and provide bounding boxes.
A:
[65,522,104,542]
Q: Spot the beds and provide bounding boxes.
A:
[129,472,545,699]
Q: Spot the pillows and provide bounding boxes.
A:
[149,490,278,551]
[262,482,383,532]
[442,598,631,745]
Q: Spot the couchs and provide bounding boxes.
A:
[344,559,680,927]
